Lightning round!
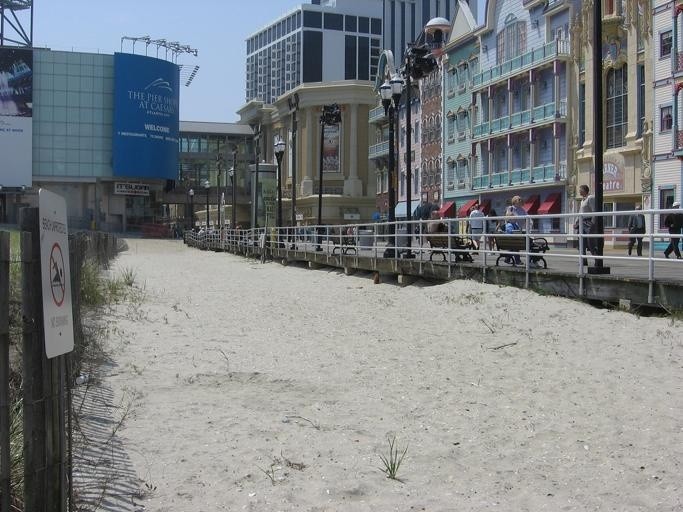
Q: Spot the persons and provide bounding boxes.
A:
[178,223,356,248]
[505,212,543,269]
[485,207,499,256]
[663,202,682,259]
[469,204,485,255]
[573,185,594,264]
[506,195,527,264]
[626,205,645,256]
[425,211,476,248]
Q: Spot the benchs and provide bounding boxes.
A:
[492,234,551,269]
[328,234,360,255]
[426,234,476,262]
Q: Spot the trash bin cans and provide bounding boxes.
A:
[358,230,375,250]
[396,229,408,247]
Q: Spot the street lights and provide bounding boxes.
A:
[401,39,440,260]
[203,180,211,229]
[272,135,285,248]
[226,166,235,230]
[315,100,343,251]
[187,188,195,230]
[121,32,201,88]
[377,71,404,260]
